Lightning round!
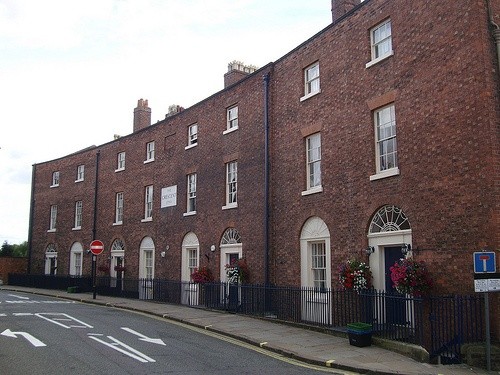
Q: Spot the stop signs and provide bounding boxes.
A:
[90,240,104,256]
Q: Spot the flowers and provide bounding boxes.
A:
[335,256,436,301]
[98,263,127,274]
[190,256,252,285]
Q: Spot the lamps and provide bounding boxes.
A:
[361,246,375,257]
[160,251,166,258]
[210,244,216,252]
[401,243,412,255]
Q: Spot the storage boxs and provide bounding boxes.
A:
[346,321,373,349]
